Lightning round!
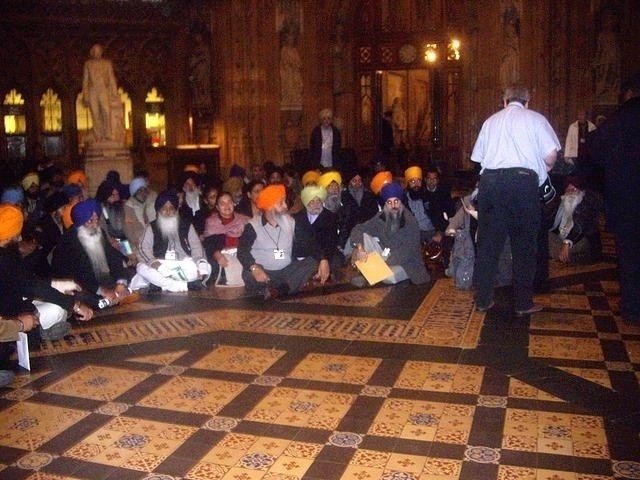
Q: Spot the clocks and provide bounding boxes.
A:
[399,43,417,63]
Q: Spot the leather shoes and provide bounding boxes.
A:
[516,303,542,316]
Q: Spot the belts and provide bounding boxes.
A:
[508,167,532,176]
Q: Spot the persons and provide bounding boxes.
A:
[310,108,343,172]
[1,166,604,390]
[277,36,304,111]
[564,109,598,175]
[470,84,563,316]
[595,114,607,127]
[188,31,213,116]
[392,96,407,144]
[591,74,639,327]
[83,45,118,142]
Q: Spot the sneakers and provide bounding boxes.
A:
[40,322,70,341]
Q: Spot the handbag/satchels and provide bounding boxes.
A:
[449,228,475,289]
[419,240,448,271]
